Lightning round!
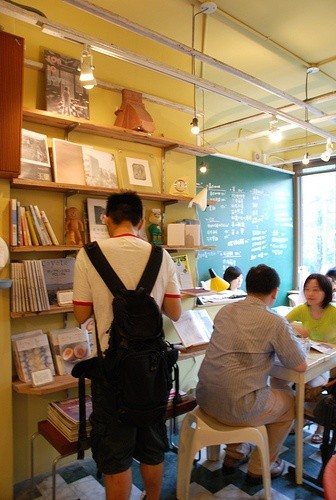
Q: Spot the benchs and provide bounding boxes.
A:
[30,354,196,499]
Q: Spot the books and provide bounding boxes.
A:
[82,197,110,245]
[44,48,91,120]
[47,394,93,443]
[11,253,76,312]
[51,138,84,186]
[166,386,188,409]
[179,288,217,297]
[161,307,215,350]
[10,315,97,383]
[82,144,119,189]
[12,127,51,182]
[9,198,59,247]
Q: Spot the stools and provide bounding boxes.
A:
[175,405,272,500]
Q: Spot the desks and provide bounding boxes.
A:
[267,340,336,485]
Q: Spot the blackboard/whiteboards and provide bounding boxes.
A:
[192,153,296,311]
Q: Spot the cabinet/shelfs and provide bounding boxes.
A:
[11,106,218,396]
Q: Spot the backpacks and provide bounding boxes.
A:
[81,240,179,432]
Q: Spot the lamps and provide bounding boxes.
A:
[320,136,334,162]
[302,152,310,165]
[76,44,97,89]
[190,118,200,135]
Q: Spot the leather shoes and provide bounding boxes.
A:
[246,457,286,486]
[222,443,256,473]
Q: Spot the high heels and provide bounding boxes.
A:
[289,421,314,435]
[311,427,324,443]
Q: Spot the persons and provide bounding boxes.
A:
[267,273,336,444]
[222,266,243,291]
[65,206,85,246]
[195,264,307,479]
[72,191,184,500]
[147,211,164,245]
